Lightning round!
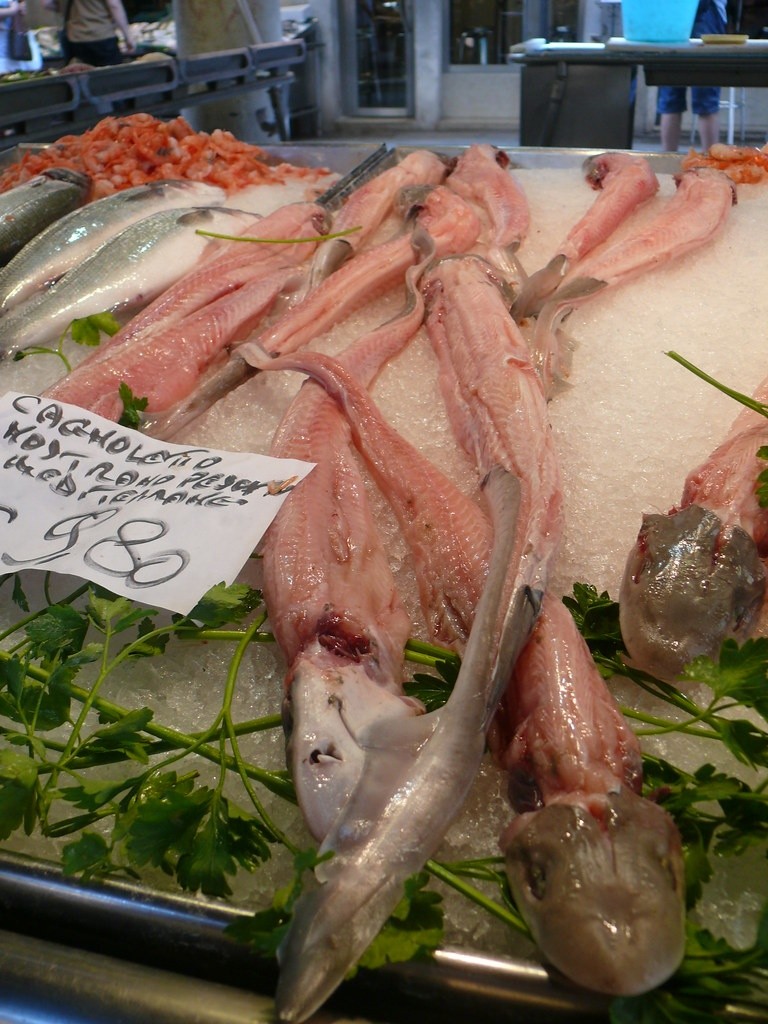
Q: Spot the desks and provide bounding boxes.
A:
[506,37,768,150]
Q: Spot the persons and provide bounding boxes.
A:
[42,0,137,124]
[0,0,29,137]
[655,0,728,153]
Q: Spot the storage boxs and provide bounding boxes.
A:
[621,0,700,43]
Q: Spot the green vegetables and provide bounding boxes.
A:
[0,227,768,1024]
